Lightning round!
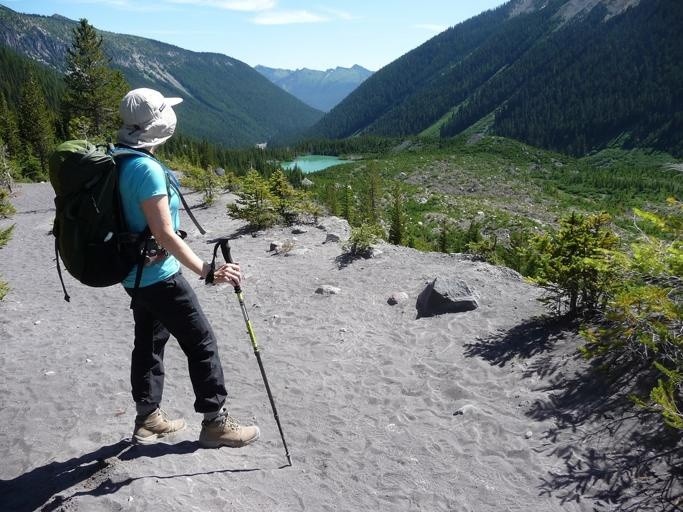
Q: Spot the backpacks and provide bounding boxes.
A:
[48,139,171,288]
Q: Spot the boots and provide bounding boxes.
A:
[199,408,261,449]
[132,407,187,445]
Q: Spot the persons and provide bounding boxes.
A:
[106,86,262,451]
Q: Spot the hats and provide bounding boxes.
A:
[116,87,183,148]
